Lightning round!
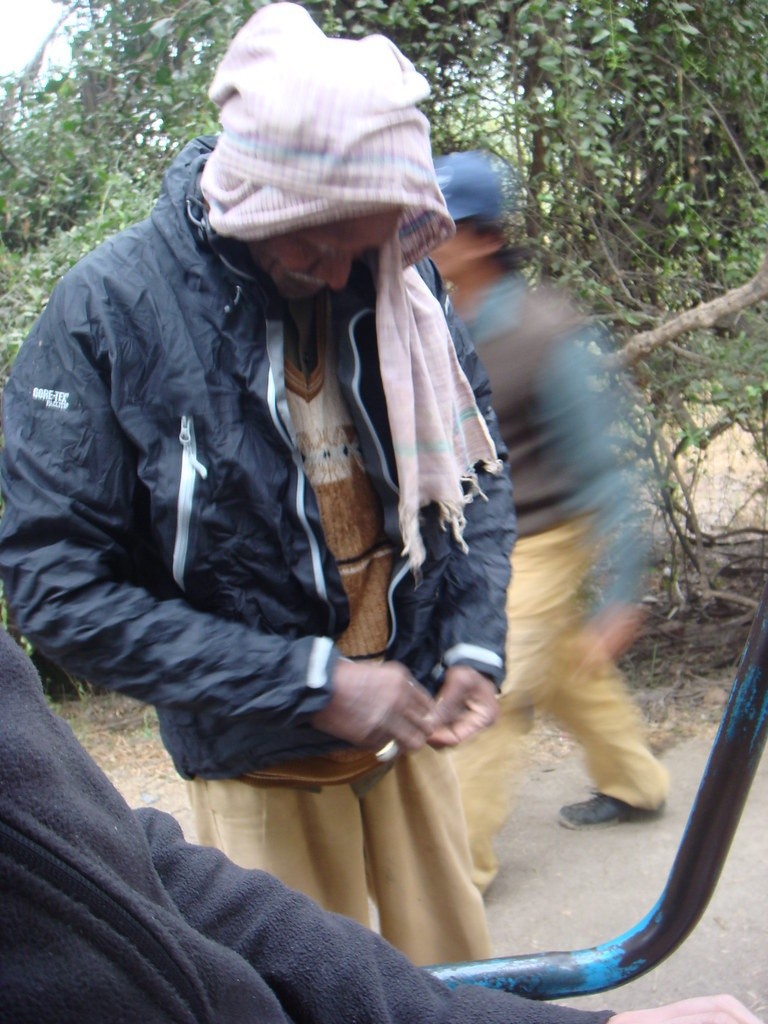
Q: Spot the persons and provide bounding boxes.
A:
[0,630,757,1024]
[0,3,517,968]
[430,155,667,895]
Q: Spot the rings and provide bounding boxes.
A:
[378,740,402,762]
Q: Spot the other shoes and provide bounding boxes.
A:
[558,793,663,830]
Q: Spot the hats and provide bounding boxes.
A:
[430,151,501,219]
